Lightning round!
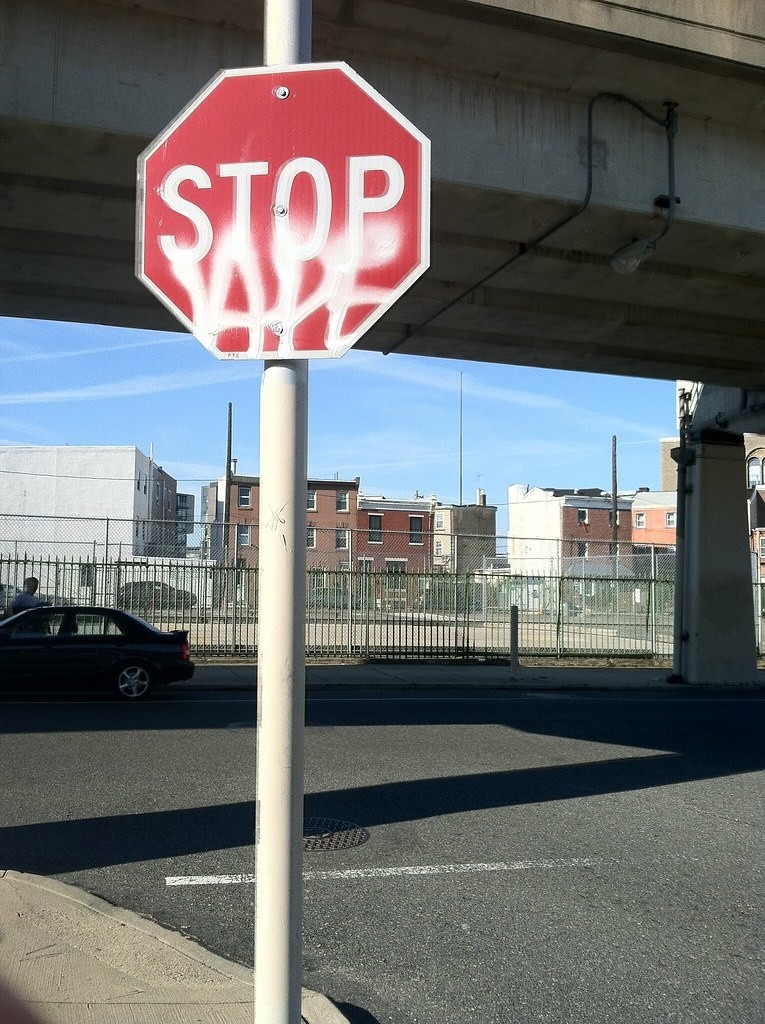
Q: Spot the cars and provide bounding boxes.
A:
[0,605,195,703]
[309,586,361,610]
[118,581,197,610]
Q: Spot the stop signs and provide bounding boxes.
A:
[130,62,433,360]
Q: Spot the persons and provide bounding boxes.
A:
[5,577,39,609]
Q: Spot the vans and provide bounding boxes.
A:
[377,588,406,609]
[411,588,465,610]
